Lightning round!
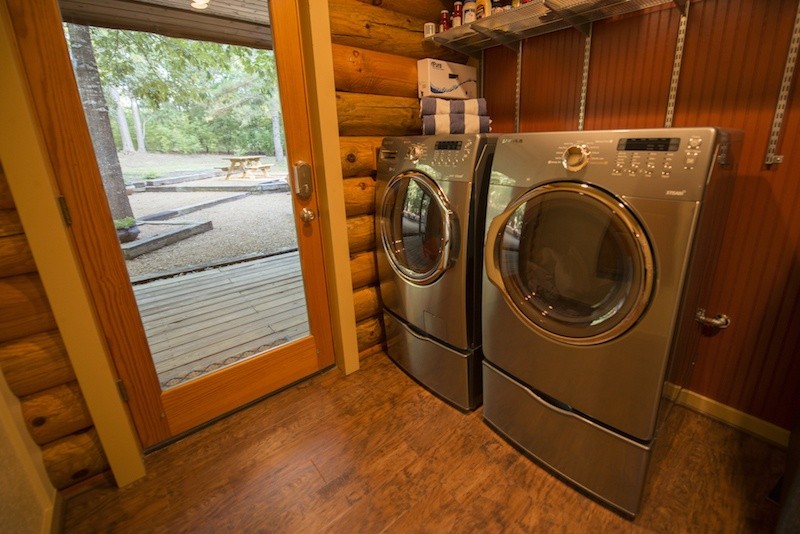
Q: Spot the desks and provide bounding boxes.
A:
[225,157,265,177]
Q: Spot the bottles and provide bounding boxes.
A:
[439,0,532,33]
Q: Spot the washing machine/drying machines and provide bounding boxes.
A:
[374,135,501,414]
[483,127,745,522]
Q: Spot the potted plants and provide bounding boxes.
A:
[114,217,140,242]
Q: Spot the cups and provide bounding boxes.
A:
[423,22,436,39]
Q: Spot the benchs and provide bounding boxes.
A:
[220,164,273,177]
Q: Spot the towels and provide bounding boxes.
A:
[417,96,487,119]
[422,115,492,135]
